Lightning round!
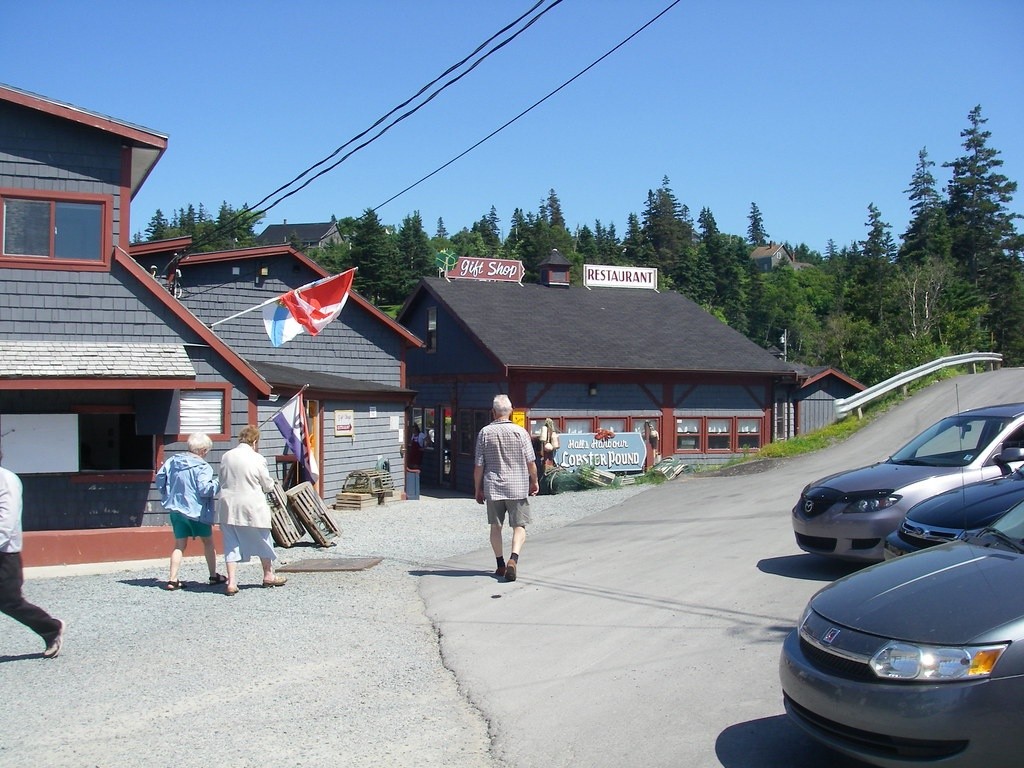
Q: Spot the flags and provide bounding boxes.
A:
[273,391,320,485]
[262,269,355,349]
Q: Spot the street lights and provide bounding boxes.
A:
[778,329,787,362]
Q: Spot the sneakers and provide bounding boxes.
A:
[44,619,64,658]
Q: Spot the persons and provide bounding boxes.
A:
[217,426,288,596]
[0,466,63,658]
[408,424,430,465]
[155,434,228,590]
[475,394,540,580]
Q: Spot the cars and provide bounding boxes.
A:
[779,501,1024,768]
[792,402,1024,563]
[886,463,1024,553]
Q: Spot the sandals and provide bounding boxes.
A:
[167,580,187,590]
[209,573,228,585]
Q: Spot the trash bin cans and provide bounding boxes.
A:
[406,466,421,500]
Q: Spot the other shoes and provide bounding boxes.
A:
[227,586,239,596]
[496,567,505,576]
[504,559,516,581]
[263,576,286,586]
